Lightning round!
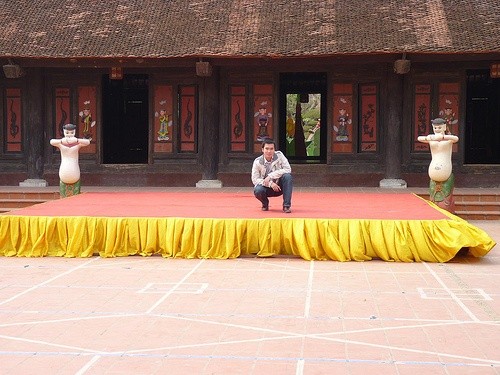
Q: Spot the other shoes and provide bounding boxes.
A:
[283,206,291,213]
[262,205,268,211]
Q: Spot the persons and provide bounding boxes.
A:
[49,124,91,200]
[417,117,459,215]
[251,139,294,213]
